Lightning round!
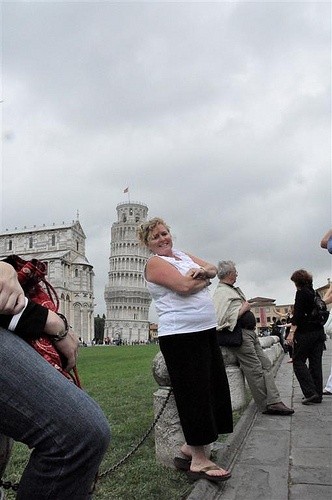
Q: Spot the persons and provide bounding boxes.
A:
[78,337,158,345]
[139,217,232,481]
[257,305,294,363]
[323,308,332,394]
[0,261,111,500]
[287,269,330,405]
[213,259,294,415]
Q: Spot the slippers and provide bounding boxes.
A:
[187,464,233,482]
[173,456,191,471]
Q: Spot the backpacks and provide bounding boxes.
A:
[297,286,329,325]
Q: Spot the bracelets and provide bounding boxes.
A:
[51,313,69,341]
[200,267,206,278]
[290,330,295,332]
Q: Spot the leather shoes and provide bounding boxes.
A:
[261,403,295,415]
[302,395,323,405]
